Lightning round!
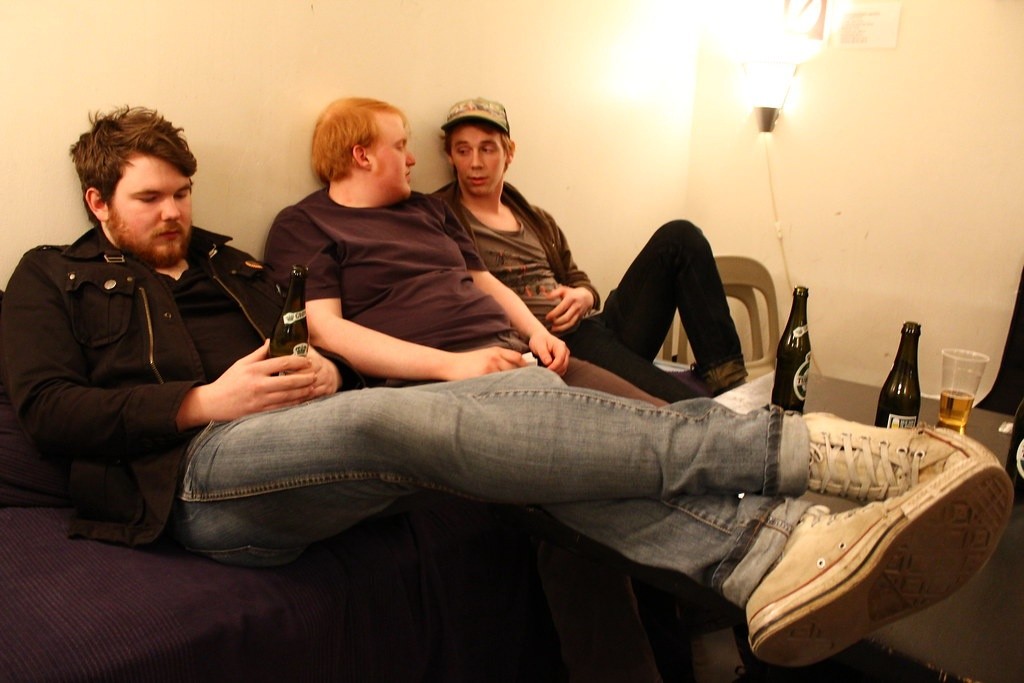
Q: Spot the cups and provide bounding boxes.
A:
[938,348,990,428]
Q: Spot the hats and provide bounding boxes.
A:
[440,97,510,137]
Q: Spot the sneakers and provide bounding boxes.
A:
[746,453,1013,666]
[800,410,1003,499]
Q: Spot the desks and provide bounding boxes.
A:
[487,362,1024,683]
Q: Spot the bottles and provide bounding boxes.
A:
[267,264,309,377]
[1006,398,1024,503]
[873,320,921,430]
[771,286,811,415]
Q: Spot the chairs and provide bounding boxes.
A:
[663,254,778,364]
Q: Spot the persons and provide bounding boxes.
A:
[433,96,749,405]
[0,106,1013,668]
[265,95,671,409]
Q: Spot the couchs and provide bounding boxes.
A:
[0,392,692,683]
